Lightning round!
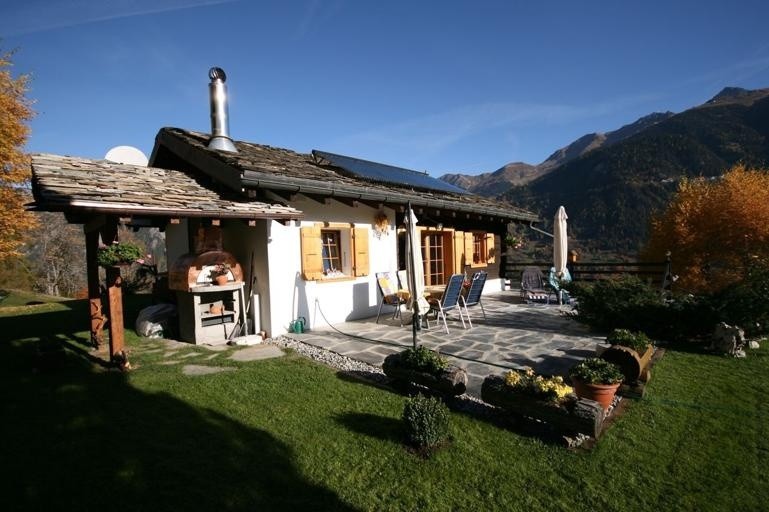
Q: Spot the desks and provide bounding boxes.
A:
[398,285,447,330]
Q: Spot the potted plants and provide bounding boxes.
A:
[570,358,626,411]
[602,329,654,381]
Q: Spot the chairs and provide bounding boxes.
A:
[519,266,555,306]
[461,271,488,328]
[426,274,467,333]
[375,272,413,327]
[396,270,408,318]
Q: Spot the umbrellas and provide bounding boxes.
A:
[402,199,431,351]
[552,205,569,306]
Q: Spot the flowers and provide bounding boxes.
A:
[500,367,574,405]
[97,240,152,268]
